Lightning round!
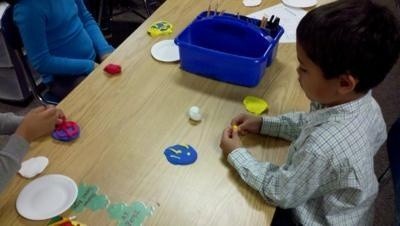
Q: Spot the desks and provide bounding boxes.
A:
[0,0,337,226]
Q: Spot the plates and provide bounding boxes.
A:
[150,38,180,62]
[281,0,318,8]
[16,173,79,220]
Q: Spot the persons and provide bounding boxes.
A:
[219,0,400,226]
[0,103,65,194]
[13,0,120,99]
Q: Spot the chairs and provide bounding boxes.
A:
[374,112,400,226]
[0,2,117,110]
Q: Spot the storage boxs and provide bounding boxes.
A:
[173,12,284,87]
